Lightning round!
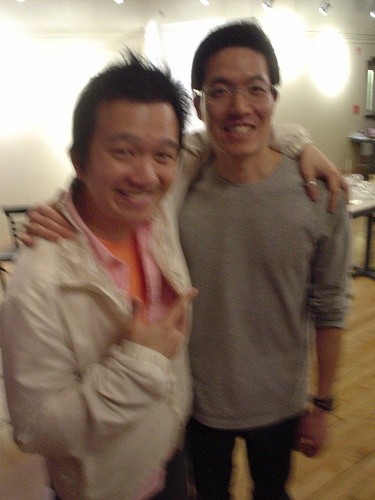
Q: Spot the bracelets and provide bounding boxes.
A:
[312,397,334,412]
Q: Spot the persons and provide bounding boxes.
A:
[20,21,355,500]
[0,46,357,500]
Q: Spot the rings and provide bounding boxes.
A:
[303,181,318,187]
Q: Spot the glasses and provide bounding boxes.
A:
[192,82,275,104]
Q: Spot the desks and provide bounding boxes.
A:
[348,130,375,171]
[337,178,375,282]
